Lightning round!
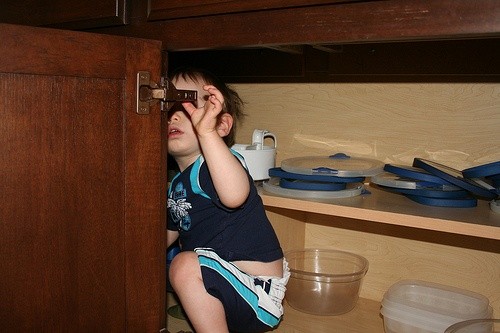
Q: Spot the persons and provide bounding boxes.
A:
[166,66,291,333]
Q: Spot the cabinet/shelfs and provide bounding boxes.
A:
[0,0,500,333]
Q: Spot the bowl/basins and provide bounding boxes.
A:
[283,248,369,316]
[444,319,500,333]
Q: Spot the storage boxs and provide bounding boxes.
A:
[380,279,494,332]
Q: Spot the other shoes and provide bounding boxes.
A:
[167,305,188,320]
